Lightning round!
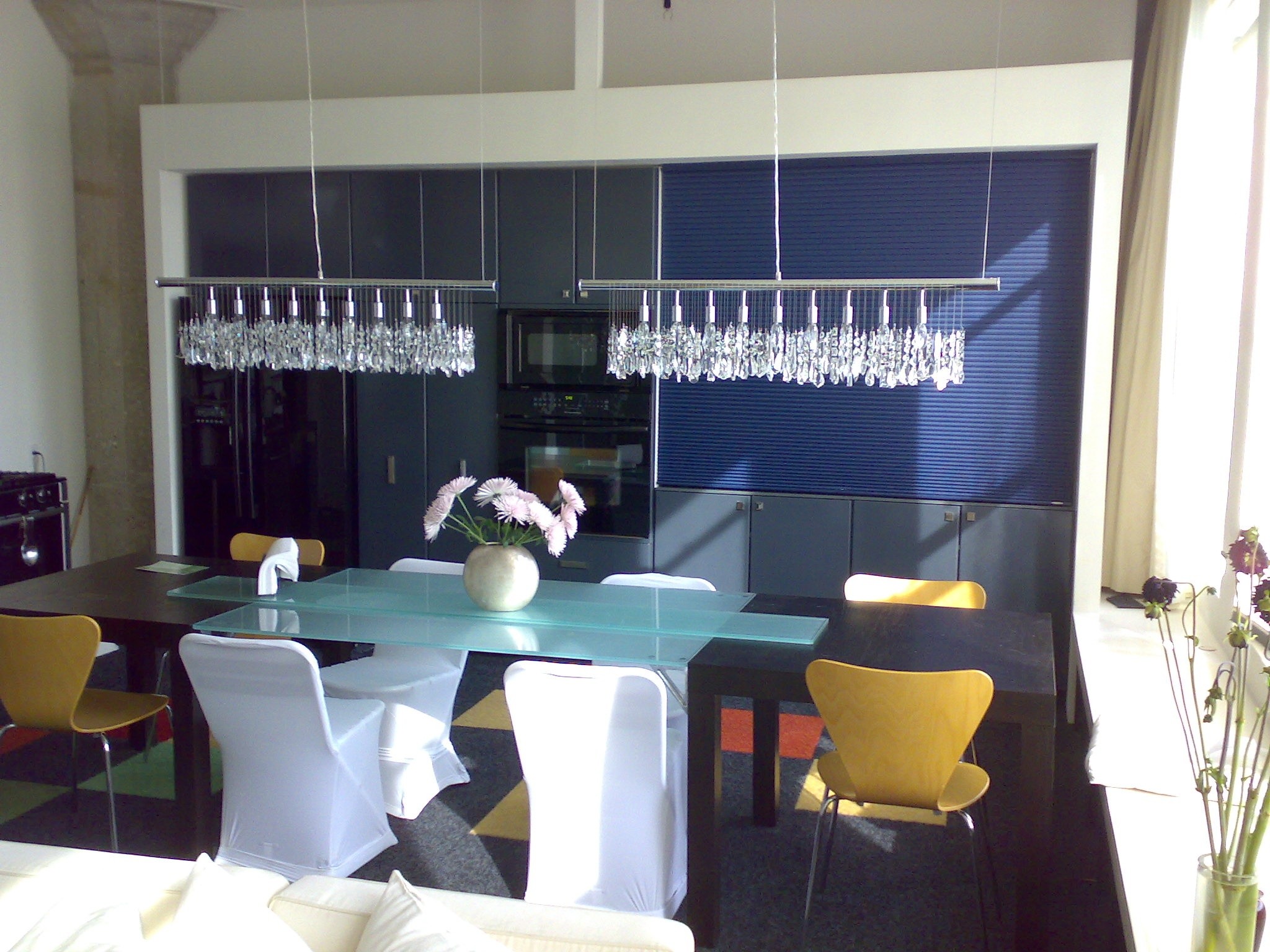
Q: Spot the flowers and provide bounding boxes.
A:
[422,476,586,558]
[1135,525,1270,869]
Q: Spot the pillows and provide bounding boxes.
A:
[145,852,311,952]
[355,870,480,952]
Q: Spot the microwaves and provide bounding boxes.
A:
[505,310,640,387]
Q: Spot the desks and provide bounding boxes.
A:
[0,553,1057,952]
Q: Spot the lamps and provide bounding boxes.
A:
[155,0,496,377]
[580,0,1002,390]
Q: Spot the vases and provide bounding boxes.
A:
[1191,854,1260,952]
[463,544,540,612]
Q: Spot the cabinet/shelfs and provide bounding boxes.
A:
[182,151,1094,699]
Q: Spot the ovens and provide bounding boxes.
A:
[498,389,655,540]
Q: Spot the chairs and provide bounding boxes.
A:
[798,659,994,944]
[142,533,325,765]
[179,633,399,880]
[503,661,687,918]
[320,558,466,737]
[0,614,169,853]
[844,573,988,760]
[591,574,717,719]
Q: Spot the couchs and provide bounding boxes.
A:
[0,839,695,952]
